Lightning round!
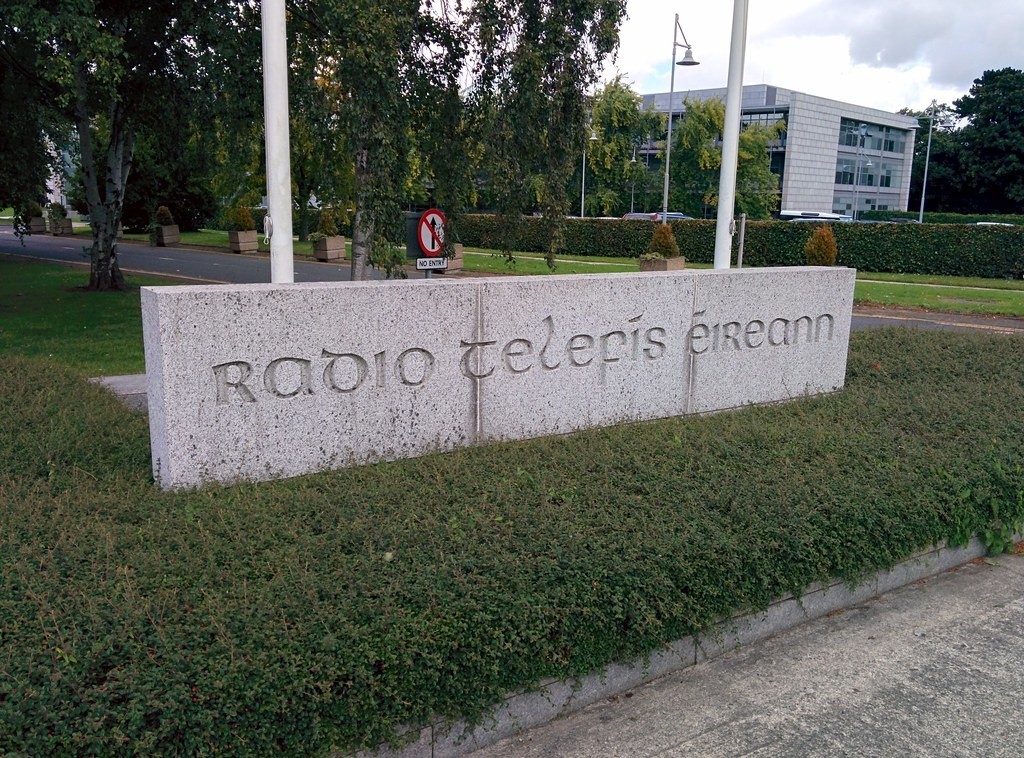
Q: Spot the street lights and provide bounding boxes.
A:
[581,124,599,218]
[853,147,872,222]
[629,141,638,215]
[663,14,701,225]
[907,100,955,224]
